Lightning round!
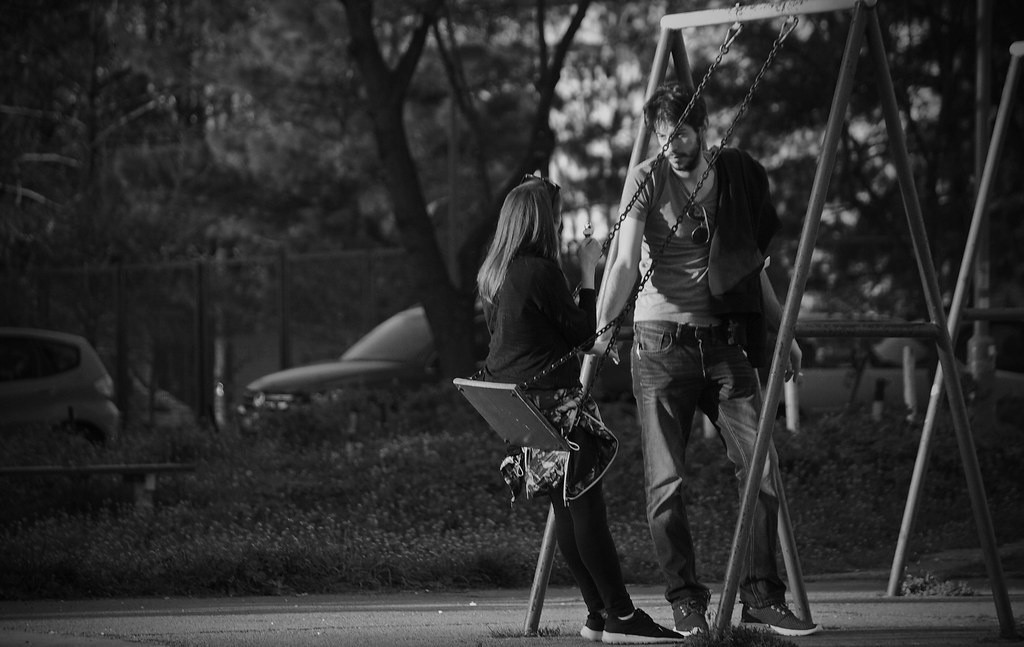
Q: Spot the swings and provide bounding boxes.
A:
[451,16,801,454]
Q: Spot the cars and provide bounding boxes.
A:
[767,316,974,429]
[236,302,493,445]
[0,328,122,496]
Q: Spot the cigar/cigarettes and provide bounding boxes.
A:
[784,368,804,377]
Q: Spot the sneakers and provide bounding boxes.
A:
[671,593,709,636]
[580,614,608,641]
[601,606,686,643]
[738,599,825,636]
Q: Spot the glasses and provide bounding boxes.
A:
[686,201,712,246]
[520,172,557,203]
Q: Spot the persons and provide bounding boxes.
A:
[596,78,823,637]
[474,174,684,644]
